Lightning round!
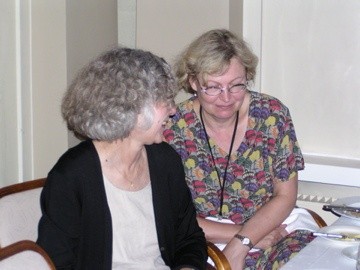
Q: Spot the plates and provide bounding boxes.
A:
[320,225,360,242]
[331,195,360,221]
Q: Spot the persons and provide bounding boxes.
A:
[37,47,208,270]
[163,29,317,270]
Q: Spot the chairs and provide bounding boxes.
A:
[0,175,325,270]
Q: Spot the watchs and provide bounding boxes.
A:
[235,234,253,250]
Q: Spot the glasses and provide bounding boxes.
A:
[194,77,249,95]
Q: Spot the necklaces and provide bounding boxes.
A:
[105,158,143,190]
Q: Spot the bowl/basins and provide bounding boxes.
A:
[341,246,360,266]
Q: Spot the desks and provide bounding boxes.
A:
[278,217,360,270]
